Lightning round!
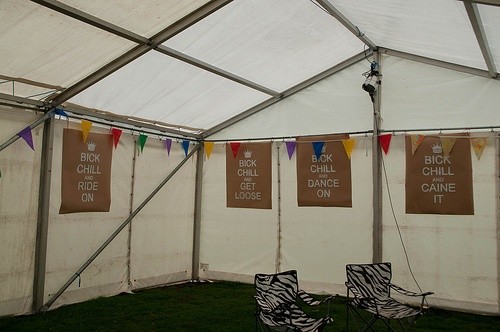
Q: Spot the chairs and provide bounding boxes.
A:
[345,262,434,331]
[254,269,338,332]
